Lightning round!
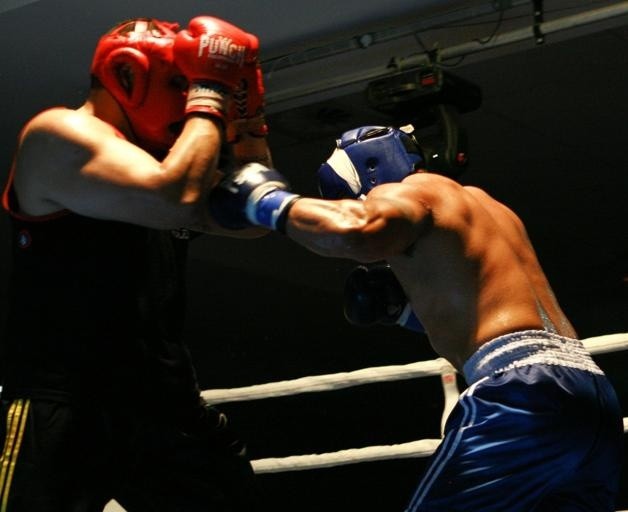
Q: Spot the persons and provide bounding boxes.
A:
[0,15,274,512]
[207,125,625,512]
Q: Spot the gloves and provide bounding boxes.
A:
[207,163,300,233]
[342,265,425,332]
[172,15,268,143]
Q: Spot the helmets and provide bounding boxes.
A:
[316,124,424,200]
[91,18,187,152]
